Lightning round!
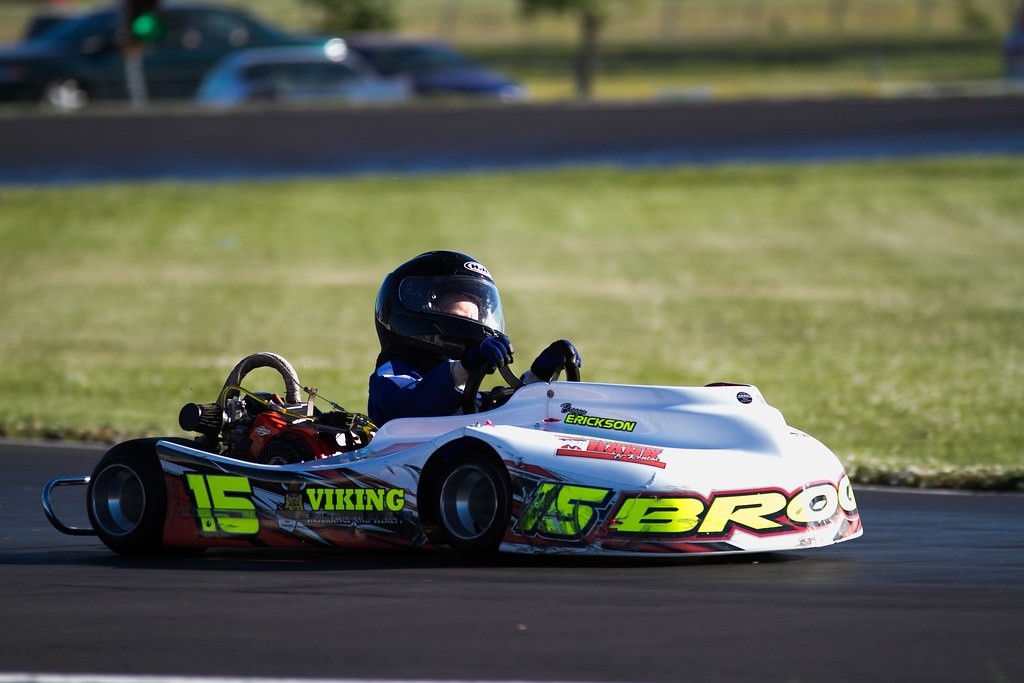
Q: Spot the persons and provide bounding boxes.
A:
[367,250,582,429]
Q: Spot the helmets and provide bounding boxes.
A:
[374,250,507,370]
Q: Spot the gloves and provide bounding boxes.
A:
[531,338,580,384]
[461,333,515,375]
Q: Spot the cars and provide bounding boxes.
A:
[0,0,330,111]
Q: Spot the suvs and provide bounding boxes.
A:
[194,37,526,100]
